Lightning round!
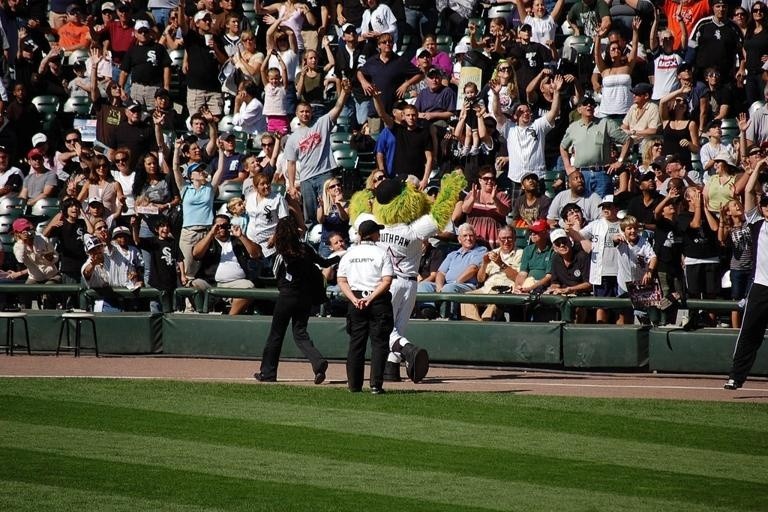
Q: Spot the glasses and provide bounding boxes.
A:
[261,143,273,146]
[500,66,511,72]
[115,158,127,163]
[752,8,763,13]
[654,143,662,146]
[375,175,386,183]
[112,85,121,89]
[66,138,79,143]
[327,183,340,189]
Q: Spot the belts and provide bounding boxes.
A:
[392,274,417,280]
[580,166,608,171]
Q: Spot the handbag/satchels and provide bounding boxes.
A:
[627,279,663,309]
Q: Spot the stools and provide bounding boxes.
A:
[0,310,33,356]
[55,310,98,359]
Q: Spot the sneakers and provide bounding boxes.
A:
[314,360,328,384]
[346,382,361,392]
[254,373,276,382]
[460,146,479,156]
[422,307,439,318]
[711,320,730,328]
[372,387,384,394]
[724,379,737,390]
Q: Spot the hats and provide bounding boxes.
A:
[579,94,595,104]
[341,23,356,36]
[126,87,171,110]
[635,157,666,185]
[218,133,235,143]
[194,11,211,22]
[528,220,568,242]
[746,145,761,156]
[12,218,32,233]
[416,46,441,77]
[676,61,691,73]
[713,153,736,167]
[187,164,207,177]
[705,119,722,130]
[521,172,538,183]
[27,133,47,158]
[517,24,531,33]
[111,225,131,238]
[359,220,385,236]
[629,83,653,95]
[598,195,619,207]
[424,185,441,195]
[482,177,495,180]
[65,1,151,32]
[84,238,105,252]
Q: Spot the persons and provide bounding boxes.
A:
[1,1,767,330]
[722,158,767,390]
[253,217,345,385]
[336,219,393,395]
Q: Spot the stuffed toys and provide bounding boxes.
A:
[348,167,467,383]
